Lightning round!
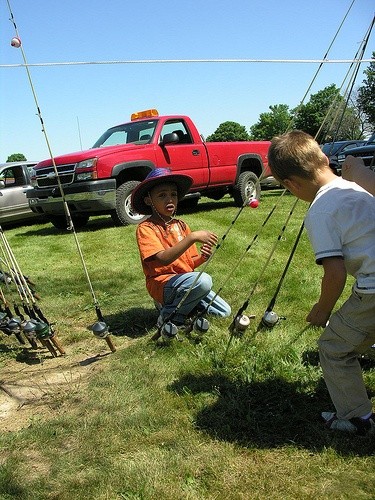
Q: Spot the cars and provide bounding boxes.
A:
[1,160,49,224]
[321,140,366,174]
[338,133,375,171]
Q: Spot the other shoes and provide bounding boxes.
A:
[320,410,375,433]
[157,315,187,334]
[190,305,205,321]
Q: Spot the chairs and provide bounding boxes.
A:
[140,134,151,140]
[162,132,179,143]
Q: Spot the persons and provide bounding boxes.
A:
[130,168,233,330]
[267,129,375,438]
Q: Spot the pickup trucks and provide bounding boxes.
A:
[26,109,272,227]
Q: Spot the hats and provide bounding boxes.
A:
[131,168,193,216]
